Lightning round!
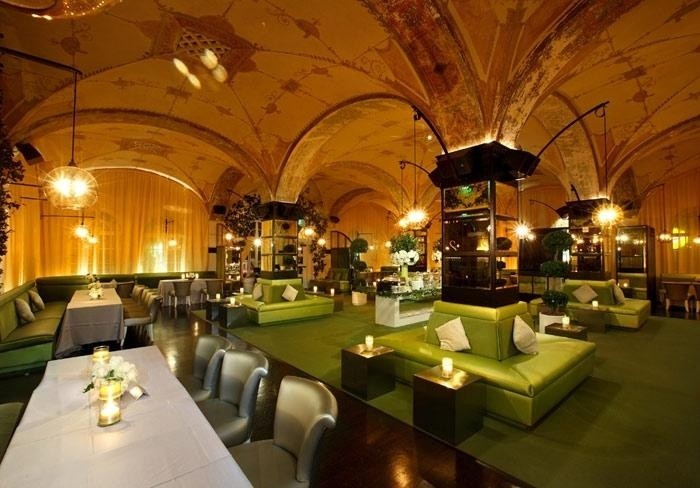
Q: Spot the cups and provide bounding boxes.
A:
[365,334,373,352]
[93,345,122,427]
[592,300,599,307]
[228,275,242,281]
[313,285,335,296]
[562,316,570,328]
[181,273,199,279]
[215,287,244,306]
[441,356,453,380]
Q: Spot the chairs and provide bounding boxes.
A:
[176,335,232,403]
[197,349,269,447]
[120,285,164,350]
[0,402,24,465]
[227,375,338,488]
[181,280,209,318]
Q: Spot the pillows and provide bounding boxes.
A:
[28,289,46,311]
[15,298,36,322]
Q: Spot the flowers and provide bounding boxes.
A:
[387,233,420,266]
[81,355,137,394]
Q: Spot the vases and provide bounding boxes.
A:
[400,266,408,280]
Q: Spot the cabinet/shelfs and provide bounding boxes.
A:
[216,222,243,294]
[441,177,655,315]
[260,219,298,279]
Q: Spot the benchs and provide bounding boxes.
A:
[227,278,334,326]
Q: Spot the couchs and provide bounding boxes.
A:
[36,271,216,302]
[0,279,66,374]
